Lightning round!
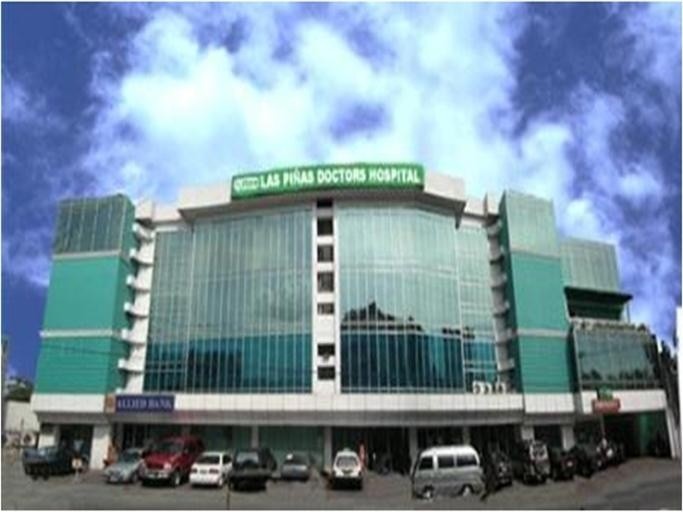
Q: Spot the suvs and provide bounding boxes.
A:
[137,434,205,487]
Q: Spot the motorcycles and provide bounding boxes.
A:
[512,437,623,487]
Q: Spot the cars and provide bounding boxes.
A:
[330,449,364,490]
[188,450,230,488]
[102,447,144,484]
[480,449,515,490]
[280,450,312,482]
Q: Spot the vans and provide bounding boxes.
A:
[20,425,92,482]
[229,447,277,490]
[410,445,486,503]
